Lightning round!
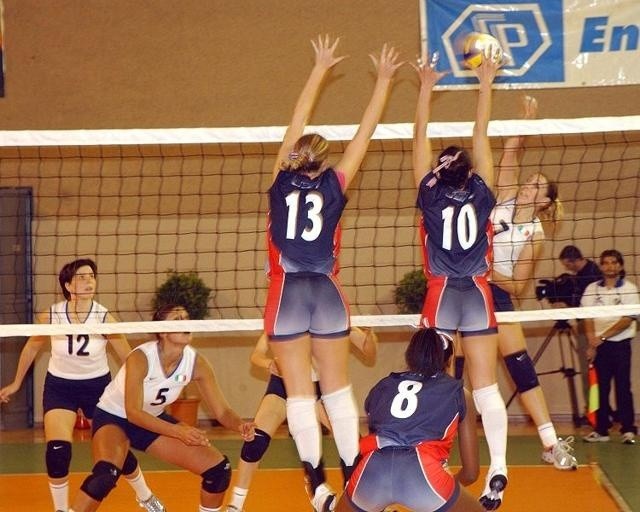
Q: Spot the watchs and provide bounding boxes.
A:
[600,334,607,342]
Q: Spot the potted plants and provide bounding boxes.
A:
[151,267,211,426]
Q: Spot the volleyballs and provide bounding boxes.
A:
[464,33,501,69]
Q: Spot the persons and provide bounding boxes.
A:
[407,42,508,512]
[0,257,170,510]
[262,31,409,511]
[581,248,639,444]
[65,272,260,511]
[228,324,378,512]
[452,92,580,472]
[334,328,493,512]
[559,238,602,313]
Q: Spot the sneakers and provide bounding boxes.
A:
[621,432,635,443]
[540,443,577,471]
[477,464,509,510]
[582,431,611,443]
[304,477,337,512]
[135,492,166,512]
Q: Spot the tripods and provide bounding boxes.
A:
[504,321,623,434]
